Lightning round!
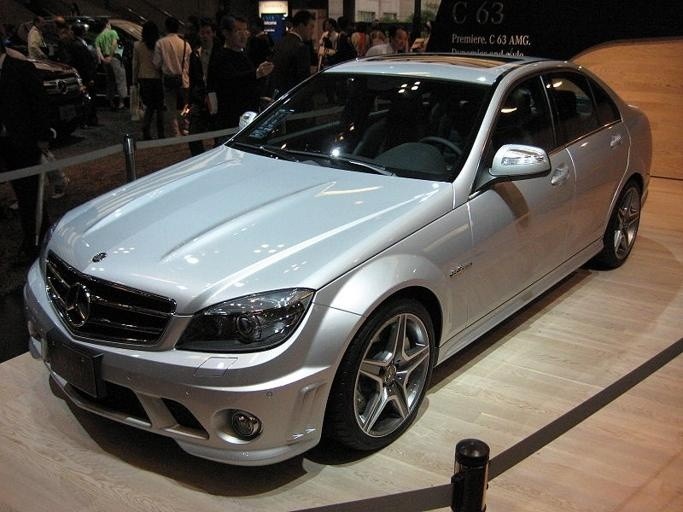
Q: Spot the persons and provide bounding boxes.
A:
[0,11,409,259]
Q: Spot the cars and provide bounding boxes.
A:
[23,51,652,468]
[0,15,165,138]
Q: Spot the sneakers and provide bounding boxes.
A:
[9,201,19,210]
[51,189,65,199]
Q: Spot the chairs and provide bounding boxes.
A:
[352,90,536,158]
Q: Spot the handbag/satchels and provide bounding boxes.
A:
[138,77,164,106]
[162,74,182,89]
